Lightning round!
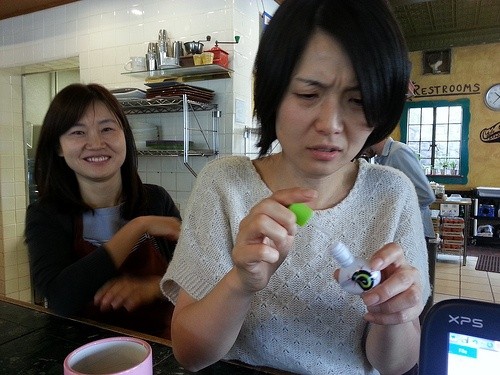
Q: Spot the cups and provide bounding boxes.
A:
[124,56,147,72]
[64,337,153,375]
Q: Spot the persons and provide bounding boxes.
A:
[158,0,428,375]
[368,134,439,265]
[22,83,181,341]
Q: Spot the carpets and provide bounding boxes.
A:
[475,254,500,273]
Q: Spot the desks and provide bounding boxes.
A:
[429,196,472,266]
[444,183,474,198]
[0,294,295,374]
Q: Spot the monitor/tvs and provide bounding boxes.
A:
[419,299,499,375]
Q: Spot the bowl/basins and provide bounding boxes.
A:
[184,41,204,55]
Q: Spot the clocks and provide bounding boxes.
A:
[484,83,500,111]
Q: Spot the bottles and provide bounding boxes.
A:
[288,203,382,296]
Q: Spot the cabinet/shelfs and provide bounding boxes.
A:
[115,99,221,178]
[470,185,500,247]
[440,223,465,251]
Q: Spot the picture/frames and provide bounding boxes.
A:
[423,48,451,74]
